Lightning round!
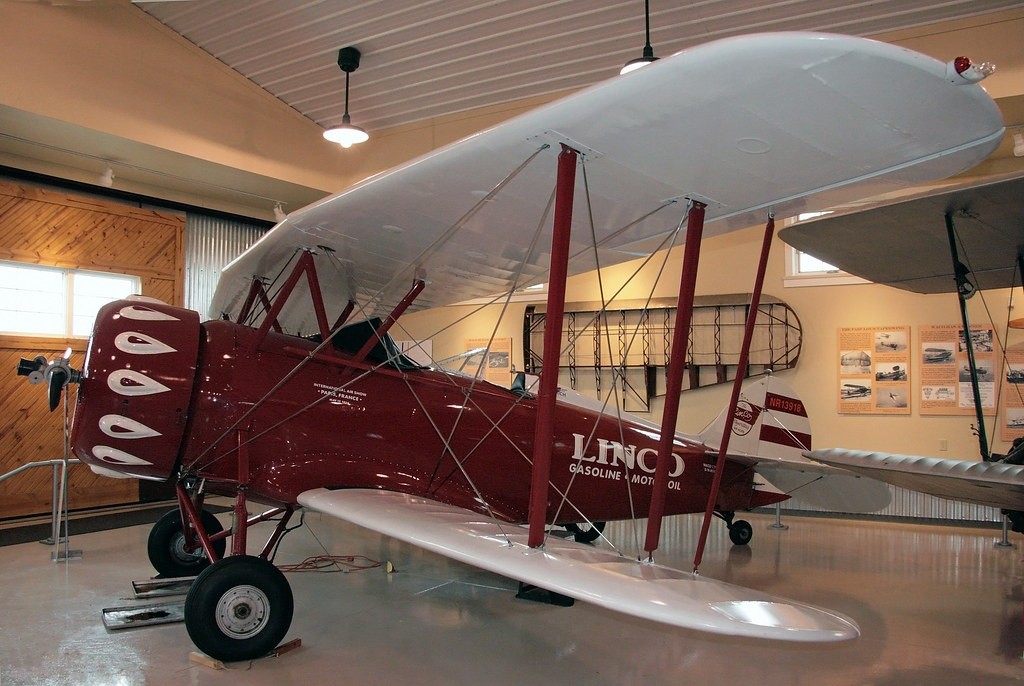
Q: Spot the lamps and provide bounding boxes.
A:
[619,1,661,75]
[324,47,369,149]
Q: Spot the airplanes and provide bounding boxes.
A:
[17,25,1024,664]
[841,341,1024,427]
